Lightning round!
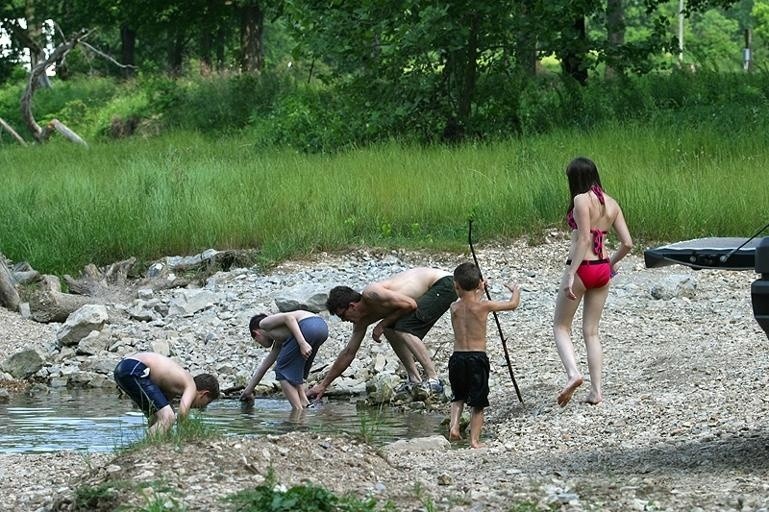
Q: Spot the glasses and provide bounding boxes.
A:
[336,308,350,319]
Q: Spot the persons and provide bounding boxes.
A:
[305,265,460,402]
[113,350,221,437]
[551,156,634,407]
[238,309,329,420]
[446,261,522,448]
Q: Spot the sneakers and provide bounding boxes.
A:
[396,377,444,393]
